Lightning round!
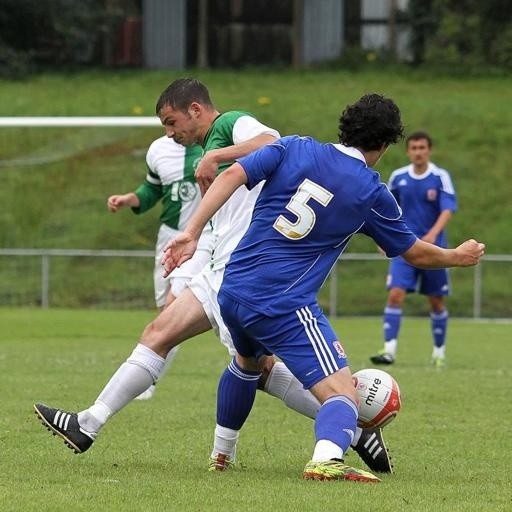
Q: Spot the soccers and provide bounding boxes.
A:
[352,369,401,429]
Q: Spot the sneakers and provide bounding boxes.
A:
[303,458,381,483]
[370,353,395,365]
[355,428,392,474]
[206,453,242,472]
[431,355,446,367]
[33,402,97,455]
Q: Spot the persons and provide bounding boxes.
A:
[157,92,486,482]
[369,132,455,366]
[37,78,392,474]
[107,135,212,399]
